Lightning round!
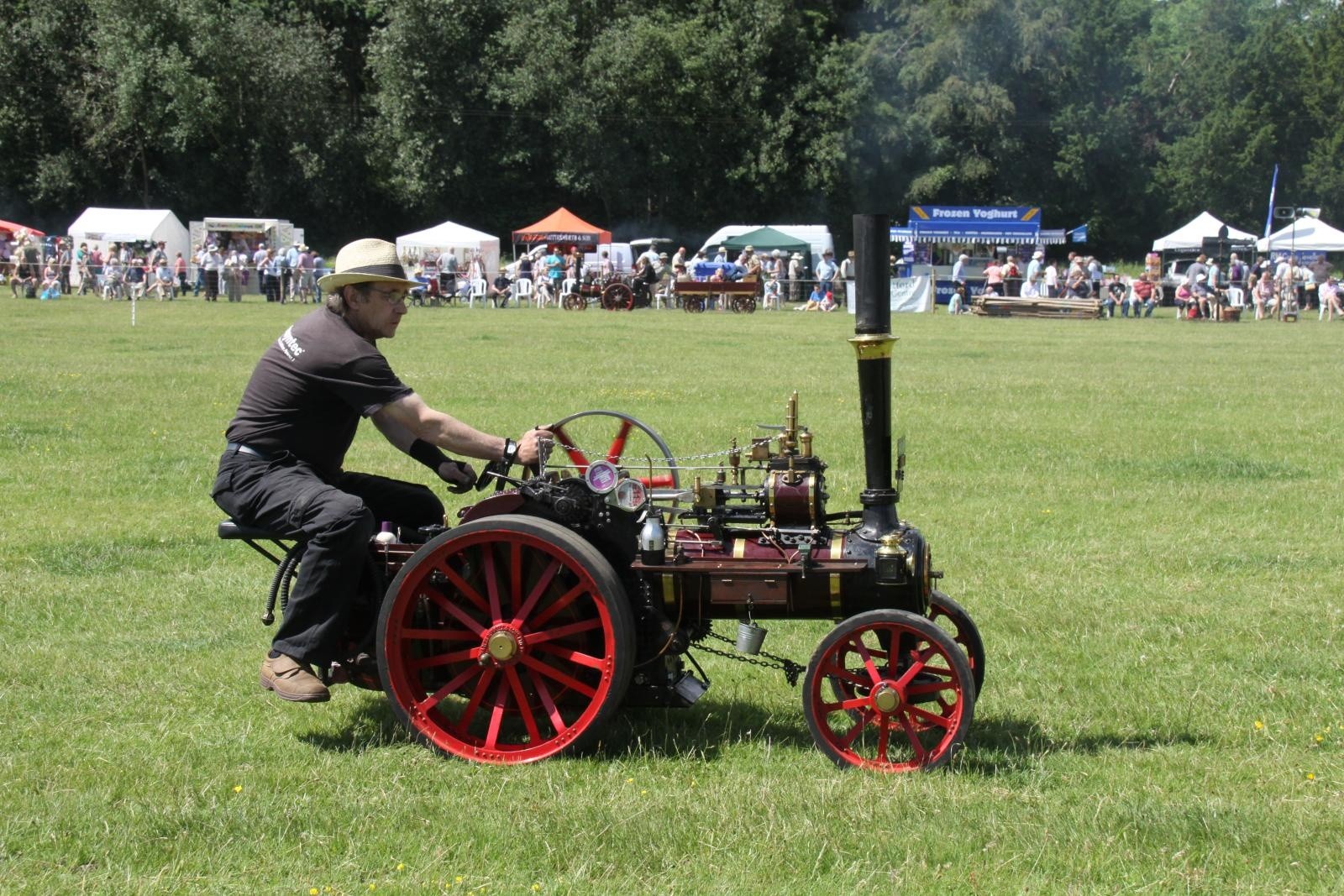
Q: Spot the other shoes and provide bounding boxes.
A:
[259,648,331,703]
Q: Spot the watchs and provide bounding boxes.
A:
[502,438,517,464]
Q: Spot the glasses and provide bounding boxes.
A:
[358,284,415,308]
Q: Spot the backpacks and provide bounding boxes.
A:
[1007,264,1017,276]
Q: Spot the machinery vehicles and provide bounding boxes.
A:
[561,250,766,314]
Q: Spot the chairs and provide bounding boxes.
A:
[10,269,175,301]
[1105,285,1153,318]
[411,278,431,308]
[1176,286,1339,321]
[468,279,487,310]
[516,278,532,308]
[764,281,785,311]
[492,283,512,308]
[537,292,554,309]
[559,278,576,309]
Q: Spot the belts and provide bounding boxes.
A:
[226,443,271,460]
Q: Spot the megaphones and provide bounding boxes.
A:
[1296,207,1321,218]
[1274,207,1295,218]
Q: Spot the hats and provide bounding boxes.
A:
[293,241,300,246]
[1196,274,1206,282]
[207,244,218,252]
[414,268,424,274]
[718,246,726,253]
[159,258,169,263]
[659,252,669,259]
[848,251,855,256]
[259,243,264,246]
[158,241,168,244]
[744,245,754,251]
[826,291,833,300]
[1196,257,1205,262]
[1274,256,1285,263]
[790,252,804,260]
[1031,251,1044,259]
[298,243,310,252]
[674,263,686,269]
[315,238,429,292]
[498,267,509,274]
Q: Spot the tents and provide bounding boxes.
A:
[1255,214,1344,259]
[1149,210,1258,279]
[67,206,192,288]
[720,225,814,303]
[395,221,501,297]
[510,207,611,278]
[0,220,45,242]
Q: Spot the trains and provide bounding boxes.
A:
[215,208,987,776]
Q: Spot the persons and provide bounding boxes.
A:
[211,235,553,705]
[889,249,1158,318]
[0,227,193,300]
[190,241,324,305]
[411,247,582,310]
[600,241,853,313]
[1174,254,1344,323]
